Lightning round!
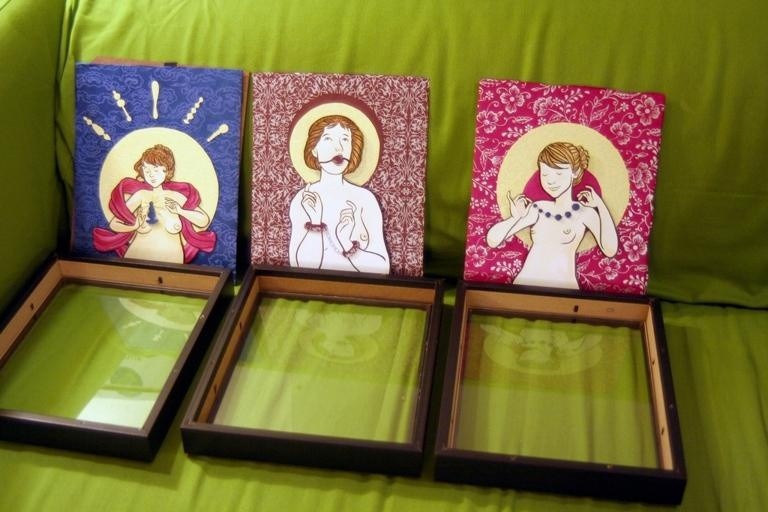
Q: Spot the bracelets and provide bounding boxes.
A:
[343,240,359,257]
[306,221,326,232]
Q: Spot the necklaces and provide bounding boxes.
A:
[533,202,581,221]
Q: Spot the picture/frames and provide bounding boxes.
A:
[178,262,450,482]
[435,278,686,507]
[0,252,238,468]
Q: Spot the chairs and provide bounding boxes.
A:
[3,0,768,511]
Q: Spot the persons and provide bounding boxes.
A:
[486,142,618,290]
[110,144,209,265]
[289,115,390,274]
